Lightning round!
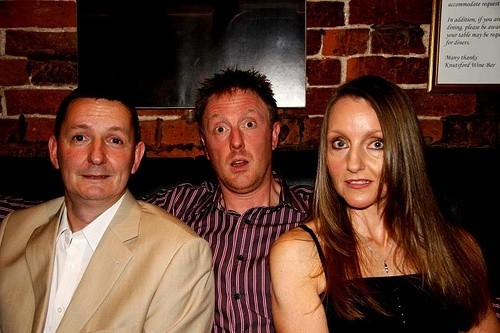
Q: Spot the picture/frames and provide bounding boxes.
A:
[427,0,500,94]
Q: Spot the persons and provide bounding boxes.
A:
[0,84,215,332]
[0,68,500,333]
[269,75,500,333]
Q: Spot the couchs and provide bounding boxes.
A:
[0,147,500,298]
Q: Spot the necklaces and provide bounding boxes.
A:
[366,244,394,273]
